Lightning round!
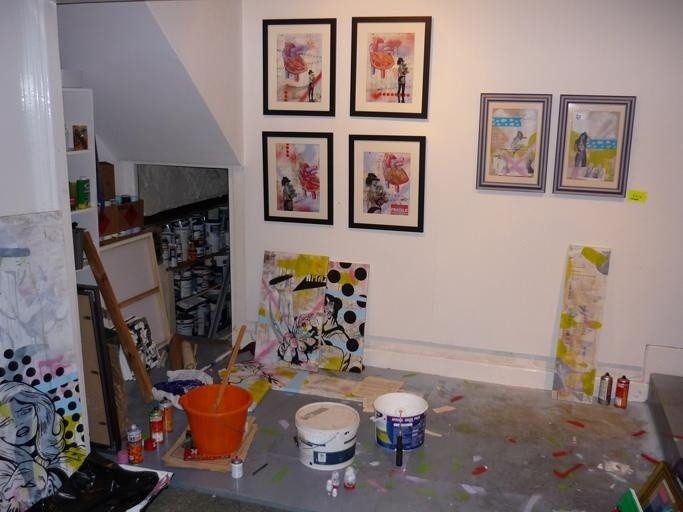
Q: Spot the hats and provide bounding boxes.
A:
[280,177,289,186]
[307,70,313,75]
[365,173,378,185]
[396,58,404,65]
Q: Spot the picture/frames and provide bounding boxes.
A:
[262,14,432,233]
[475,92,637,199]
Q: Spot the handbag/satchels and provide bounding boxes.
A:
[24,450,158,512]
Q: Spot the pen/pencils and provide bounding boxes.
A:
[252,463,268,476]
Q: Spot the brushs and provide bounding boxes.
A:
[181,340,234,371]
[396,407,407,466]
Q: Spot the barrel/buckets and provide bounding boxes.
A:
[176,315,197,337]
[179,386,253,460]
[292,401,362,473]
[370,393,431,453]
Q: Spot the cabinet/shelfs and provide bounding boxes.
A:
[61,86,101,263]
[150,195,237,340]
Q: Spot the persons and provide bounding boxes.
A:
[306,68,317,103]
[572,130,591,168]
[323,292,351,371]
[0,380,89,511]
[280,175,296,211]
[395,55,410,102]
[365,171,383,214]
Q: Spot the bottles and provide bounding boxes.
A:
[75,175,90,210]
[230,455,246,479]
[614,376,630,409]
[148,396,175,445]
[597,372,616,406]
[126,424,144,466]
[325,467,357,499]
